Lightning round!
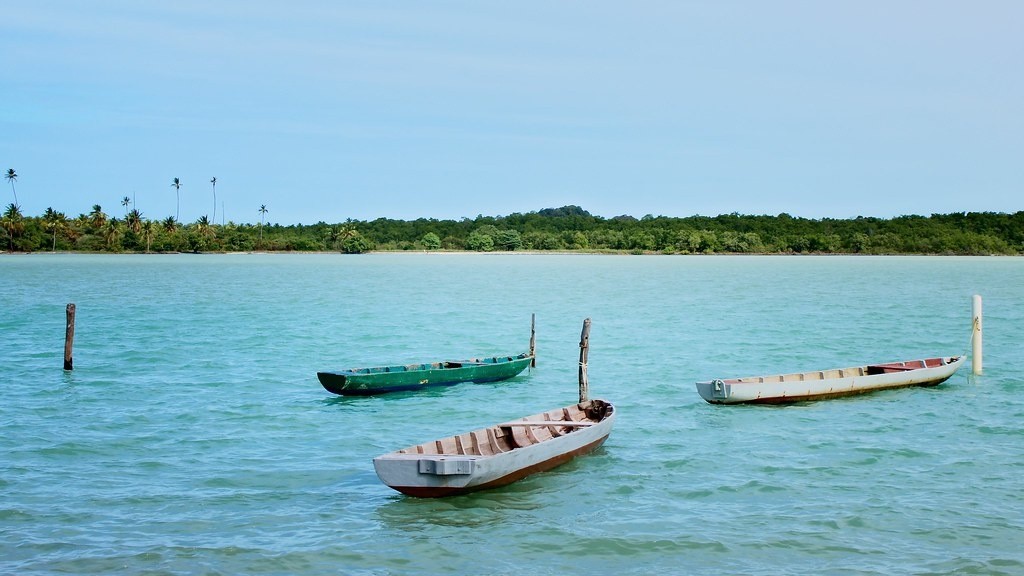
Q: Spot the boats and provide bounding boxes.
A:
[315,351,535,394]
[694,353,968,406]
[371,399,616,500]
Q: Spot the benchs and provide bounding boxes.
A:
[499,421,594,426]
[449,361,487,365]
[869,365,915,370]
